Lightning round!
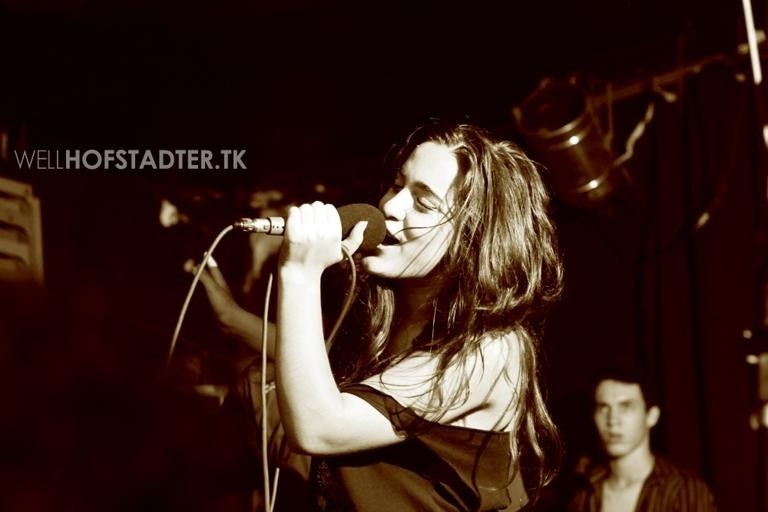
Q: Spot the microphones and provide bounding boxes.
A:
[234,203,387,252]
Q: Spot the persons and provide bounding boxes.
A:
[561,361,719,512]
[191,115,573,509]
